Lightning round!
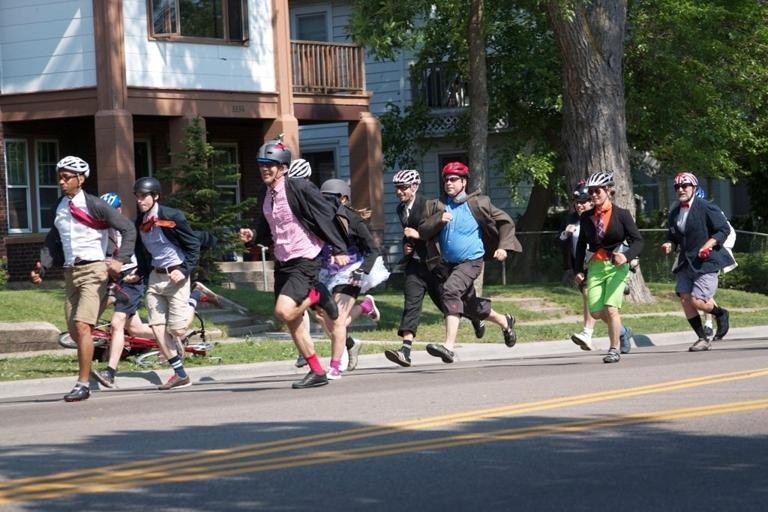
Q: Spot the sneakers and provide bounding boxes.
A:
[470,318,486,339]
[158,373,192,391]
[426,342,455,364]
[346,338,363,371]
[91,367,115,388]
[501,312,518,348]
[602,347,621,364]
[384,349,412,367]
[713,308,730,339]
[702,319,714,337]
[190,281,221,309]
[688,337,712,352]
[619,327,633,354]
[292,370,329,390]
[325,366,343,381]
[571,333,593,351]
[294,354,309,368]
[358,294,382,323]
[64,381,91,402]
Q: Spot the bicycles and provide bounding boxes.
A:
[58,312,210,368]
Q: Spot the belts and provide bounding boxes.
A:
[152,264,186,275]
[71,259,100,267]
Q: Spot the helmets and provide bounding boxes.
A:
[584,171,617,189]
[288,158,313,180]
[320,178,351,203]
[99,191,123,209]
[441,161,470,178]
[673,171,699,191]
[573,182,593,202]
[392,168,422,189]
[55,155,90,179]
[255,139,293,170]
[131,176,161,195]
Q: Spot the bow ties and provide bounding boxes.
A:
[680,203,690,209]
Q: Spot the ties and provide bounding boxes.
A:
[452,187,482,205]
[67,199,110,231]
[597,212,605,239]
[139,216,177,233]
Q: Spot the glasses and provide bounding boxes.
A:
[674,183,692,191]
[58,174,77,181]
[575,199,587,205]
[587,188,601,196]
[444,176,462,183]
[258,162,276,168]
[395,185,410,190]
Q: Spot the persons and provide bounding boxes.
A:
[239,139,353,389]
[320,177,381,380]
[90,191,184,389]
[29,154,137,402]
[669,183,739,336]
[573,170,646,363]
[285,157,365,371]
[660,171,736,353]
[555,176,634,352]
[122,175,220,391]
[383,169,486,367]
[418,160,524,364]
